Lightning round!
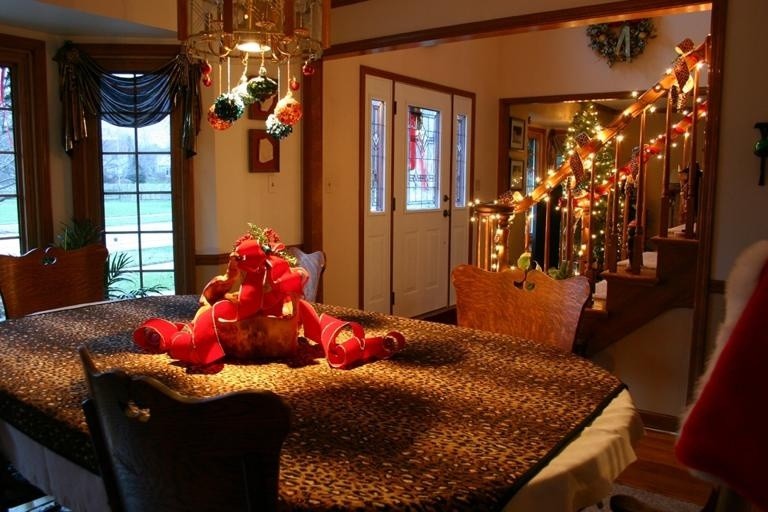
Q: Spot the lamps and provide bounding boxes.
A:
[183,0,326,140]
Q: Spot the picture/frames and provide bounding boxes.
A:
[508,118,525,150]
[248,129,279,173]
[509,158,524,190]
[247,73,280,121]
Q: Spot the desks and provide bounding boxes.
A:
[1,294,642,511]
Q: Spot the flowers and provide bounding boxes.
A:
[586,18,656,69]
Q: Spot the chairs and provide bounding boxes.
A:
[286,248,326,304]
[0,243,109,321]
[80,348,286,511]
[452,263,590,351]
